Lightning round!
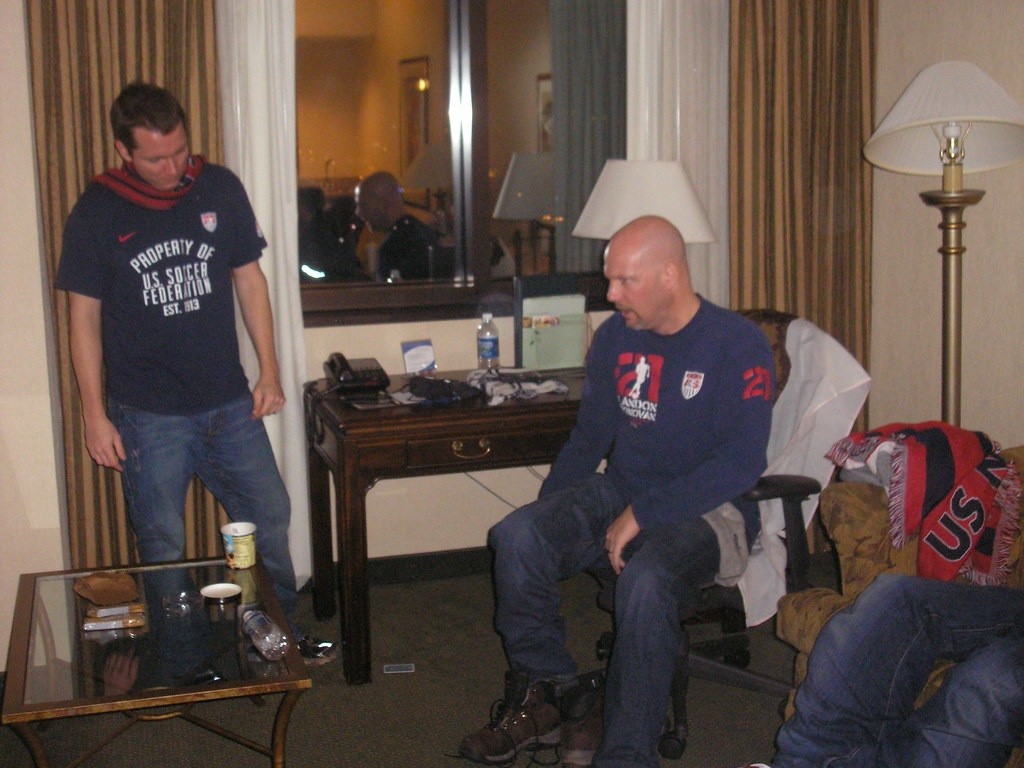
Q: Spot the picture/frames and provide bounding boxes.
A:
[537,72,554,153]
[399,56,429,147]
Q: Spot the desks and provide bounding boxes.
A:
[303,367,616,685]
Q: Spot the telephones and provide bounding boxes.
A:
[322,352,391,396]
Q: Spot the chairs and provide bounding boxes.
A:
[569,310,872,759]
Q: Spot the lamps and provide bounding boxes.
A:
[862,60,1024,427]
[400,143,452,231]
[493,152,556,272]
[570,158,715,244]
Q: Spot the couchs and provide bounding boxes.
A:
[776,444,1024,768]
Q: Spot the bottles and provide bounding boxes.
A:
[239,609,290,661]
[476,313,499,371]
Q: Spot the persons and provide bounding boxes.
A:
[102,643,141,699]
[298,172,454,285]
[55,81,339,688]
[746,571,1024,768]
[459,215,779,768]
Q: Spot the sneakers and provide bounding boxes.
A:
[458,670,561,764]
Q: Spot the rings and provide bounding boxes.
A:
[608,550,612,553]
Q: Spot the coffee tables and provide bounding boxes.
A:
[2,557,311,768]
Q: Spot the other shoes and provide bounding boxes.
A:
[139,670,194,720]
[297,635,337,659]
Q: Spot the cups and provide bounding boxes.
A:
[219,521,256,569]
[200,584,242,641]
